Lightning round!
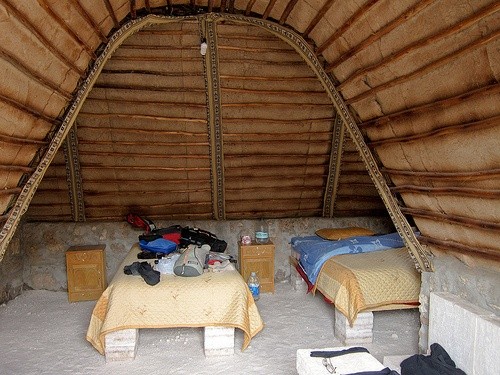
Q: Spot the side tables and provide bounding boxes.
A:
[65,245,106,302]
[238,239,275,295]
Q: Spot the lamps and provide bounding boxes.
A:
[200,37,207,55]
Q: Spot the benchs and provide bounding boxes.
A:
[383,292,500,375]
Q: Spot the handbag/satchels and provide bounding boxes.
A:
[400,343,466,375]
[127,212,156,235]
[138,224,227,277]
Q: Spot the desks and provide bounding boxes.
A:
[296,345,386,375]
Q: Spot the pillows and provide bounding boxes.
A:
[315,227,377,241]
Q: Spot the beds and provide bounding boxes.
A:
[289,235,419,344]
[88,242,264,357]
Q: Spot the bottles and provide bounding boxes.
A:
[245,272,260,301]
[255,217,269,244]
